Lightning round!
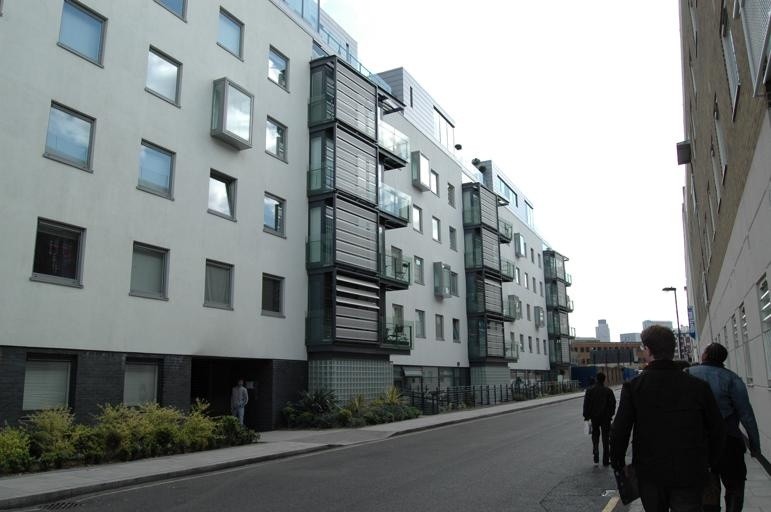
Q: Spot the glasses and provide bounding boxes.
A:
[639,342,647,350]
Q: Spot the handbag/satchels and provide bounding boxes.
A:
[614,463,640,506]
[587,422,592,435]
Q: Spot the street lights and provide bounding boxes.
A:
[662,287,682,360]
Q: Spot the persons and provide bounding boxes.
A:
[683,342,761,512]
[583,372,616,467]
[608,325,728,512]
[230,377,249,425]
[674,360,691,371]
